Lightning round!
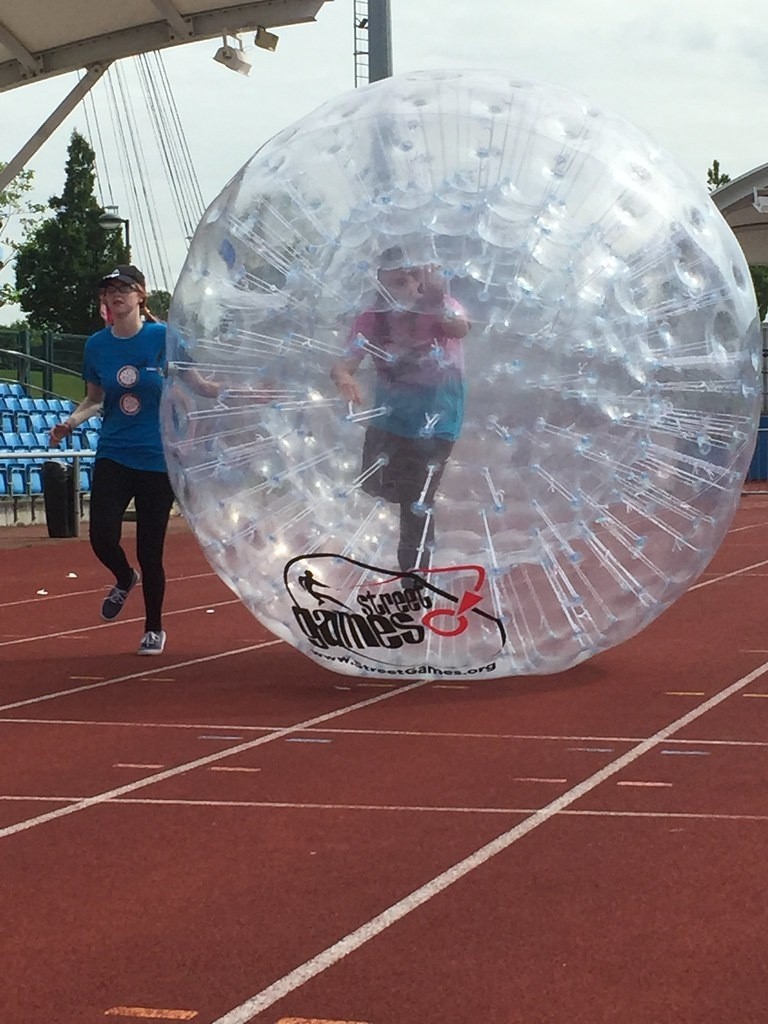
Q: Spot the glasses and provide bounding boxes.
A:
[104,284,138,293]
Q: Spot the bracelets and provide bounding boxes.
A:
[63,422,73,435]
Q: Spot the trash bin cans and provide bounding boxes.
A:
[41,460,74,537]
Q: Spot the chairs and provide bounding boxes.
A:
[1,383,104,496]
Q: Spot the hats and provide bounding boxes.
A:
[100,265,145,286]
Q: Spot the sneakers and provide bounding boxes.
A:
[136,629,166,656]
[100,568,140,621]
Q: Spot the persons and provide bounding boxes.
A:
[49,264,273,656]
[330,243,473,604]
